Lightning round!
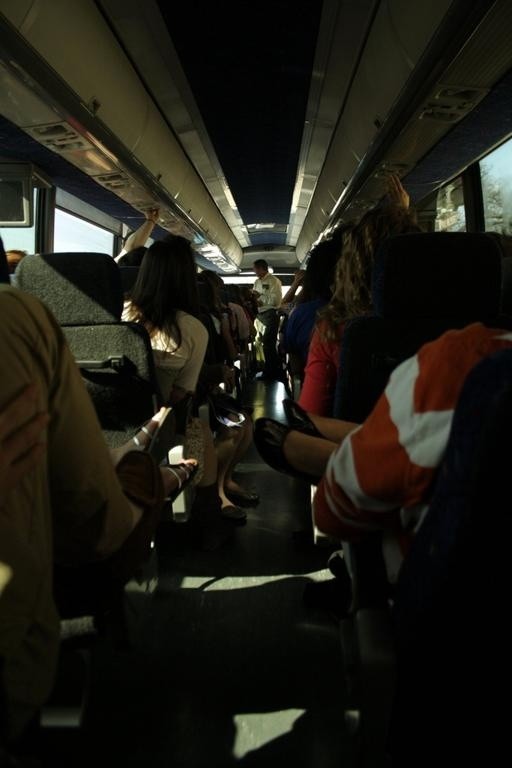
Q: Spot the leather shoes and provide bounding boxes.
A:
[282,398,326,440]
[225,485,260,503]
[254,416,318,489]
[115,449,166,567]
[217,503,248,522]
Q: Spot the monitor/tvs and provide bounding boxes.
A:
[1,163,33,229]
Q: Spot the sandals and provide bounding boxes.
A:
[159,456,199,507]
[133,403,174,457]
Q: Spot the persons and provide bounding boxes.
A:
[1,175,512,768]
